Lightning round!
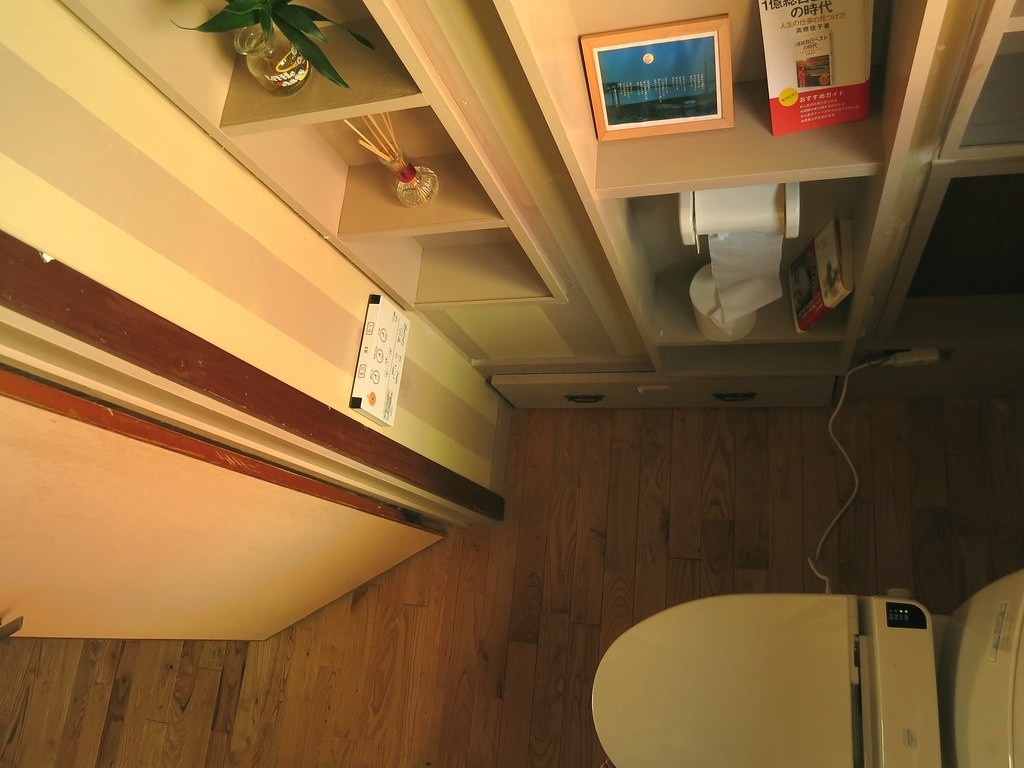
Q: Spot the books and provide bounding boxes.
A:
[788,217,854,335]
[758,0,873,138]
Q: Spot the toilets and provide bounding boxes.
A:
[591,569,1024,768]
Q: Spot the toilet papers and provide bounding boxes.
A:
[679,182,800,246]
[689,264,757,342]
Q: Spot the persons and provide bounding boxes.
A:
[791,266,812,312]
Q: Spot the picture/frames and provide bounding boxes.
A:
[581,12,735,142]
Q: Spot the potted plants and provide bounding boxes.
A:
[168,0,378,98]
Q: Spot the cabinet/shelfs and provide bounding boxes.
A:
[62,0,1024,407]
